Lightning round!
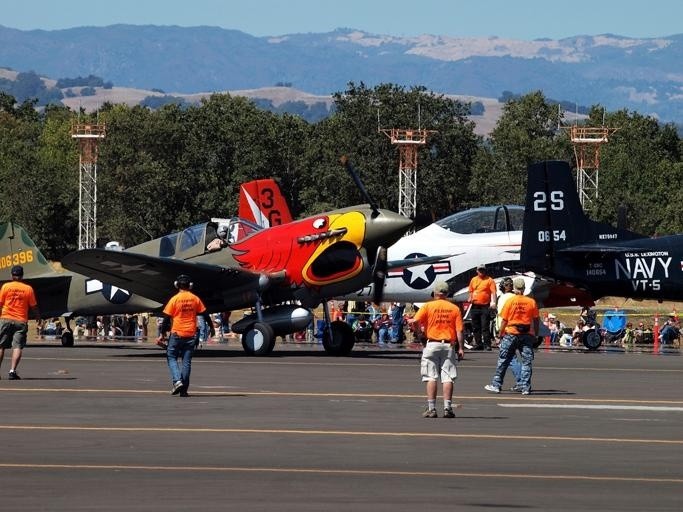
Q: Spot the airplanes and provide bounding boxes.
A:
[230,177,607,350]
[515,161,683,302]
[0,152,413,358]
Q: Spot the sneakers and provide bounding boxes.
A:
[170,380,184,396]
[421,407,437,419]
[471,343,484,351]
[483,384,500,394]
[485,343,492,352]
[7,370,21,380]
[442,407,456,418]
[510,385,532,396]
[179,393,188,397]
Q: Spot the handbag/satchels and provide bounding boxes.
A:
[514,324,530,333]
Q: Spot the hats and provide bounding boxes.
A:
[11,266,23,276]
[513,278,525,289]
[477,263,485,270]
[434,281,449,294]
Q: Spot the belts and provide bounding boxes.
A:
[428,338,450,343]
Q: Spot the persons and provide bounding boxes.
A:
[494,276,523,392]
[35,313,166,340]
[402,280,467,420]
[207,226,236,251]
[0,264,43,378]
[154,273,216,396]
[334,302,427,345]
[281,309,319,342]
[465,263,497,351]
[196,311,233,343]
[540,307,681,345]
[483,277,542,394]
